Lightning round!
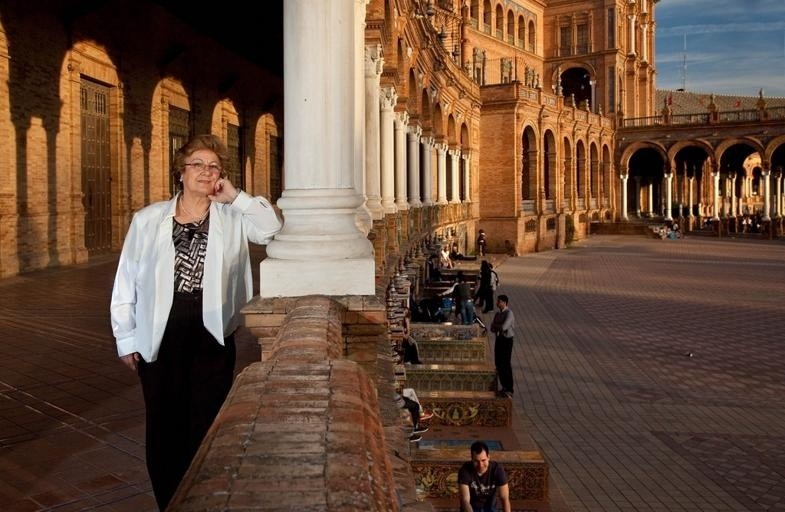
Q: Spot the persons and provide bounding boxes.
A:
[457,440,514,512]
[396,229,497,442]
[107,133,284,512]
[488,293,517,396]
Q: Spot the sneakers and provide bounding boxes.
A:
[409,426,428,442]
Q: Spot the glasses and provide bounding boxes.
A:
[185,159,222,172]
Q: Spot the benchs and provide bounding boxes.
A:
[406,268,548,512]
